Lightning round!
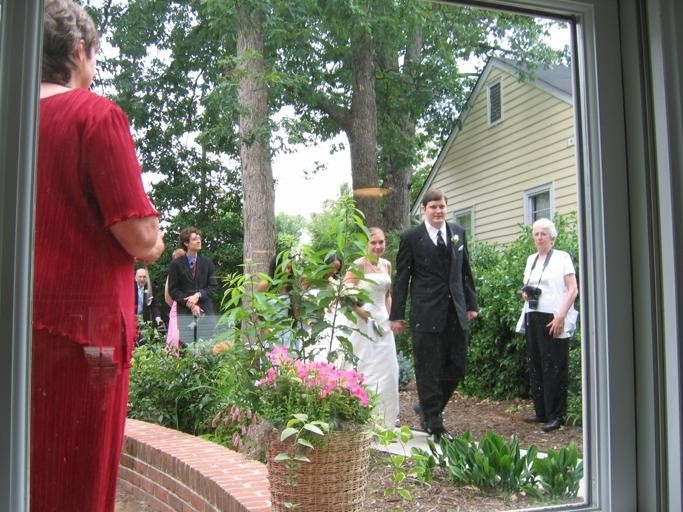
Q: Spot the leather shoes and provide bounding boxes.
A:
[524,415,564,432]
[413,401,453,443]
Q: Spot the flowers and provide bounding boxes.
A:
[218,343,373,450]
[450,232,459,249]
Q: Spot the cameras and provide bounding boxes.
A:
[521,285,541,310]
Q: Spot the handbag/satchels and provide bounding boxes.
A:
[553,309,578,339]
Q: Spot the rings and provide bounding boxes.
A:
[192,303,195,306]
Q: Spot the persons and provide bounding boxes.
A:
[249,250,296,368]
[162,246,187,361]
[27,0,165,512]
[167,227,217,358]
[291,248,346,367]
[510,215,579,434]
[339,224,402,444]
[131,266,162,349]
[386,188,480,448]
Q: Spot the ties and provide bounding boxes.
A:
[436,230,449,269]
[191,259,197,279]
